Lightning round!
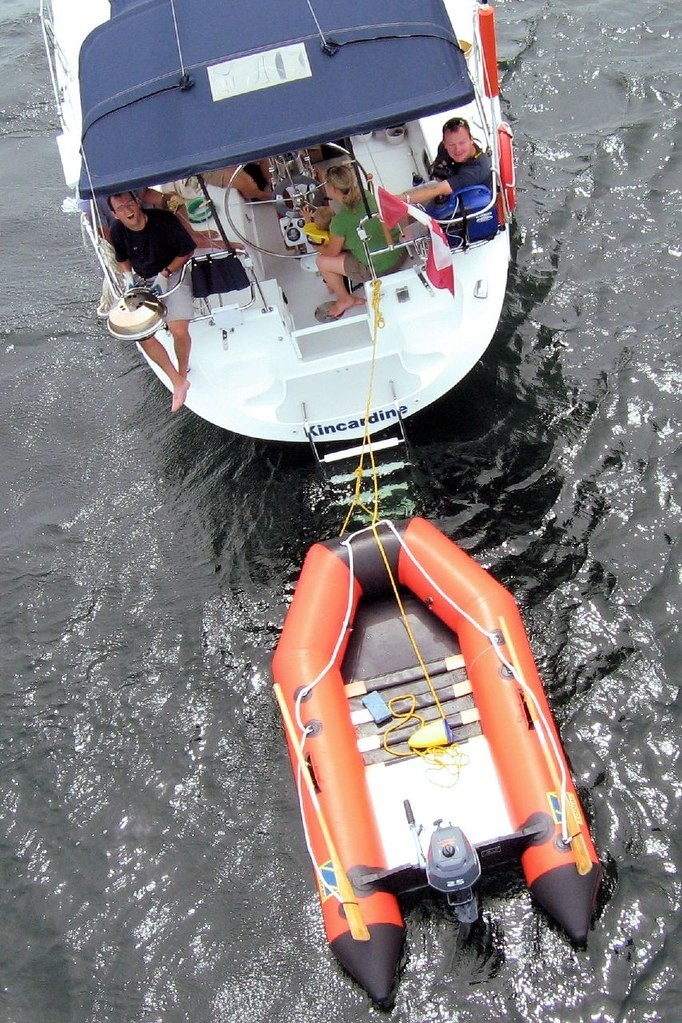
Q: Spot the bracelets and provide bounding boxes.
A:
[403,192,411,203]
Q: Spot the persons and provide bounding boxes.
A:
[196,157,274,201]
[316,165,408,317]
[394,117,499,248]
[299,206,336,245]
[83,188,250,259]
[108,192,196,412]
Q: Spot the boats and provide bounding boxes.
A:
[36,0,515,451]
[268,513,613,1017]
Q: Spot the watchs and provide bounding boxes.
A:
[162,267,172,276]
